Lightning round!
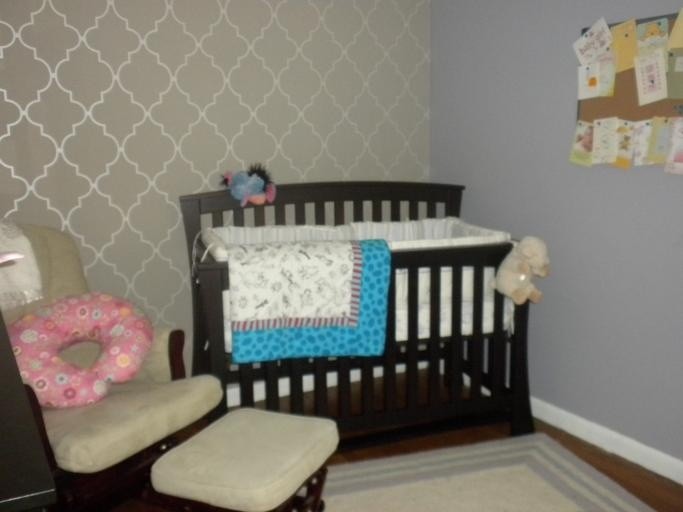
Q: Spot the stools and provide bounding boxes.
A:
[151,407,339,512]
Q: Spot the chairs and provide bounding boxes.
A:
[0,225,223,512]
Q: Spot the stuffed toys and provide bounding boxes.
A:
[493,236,549,306]
[221,164,276,206]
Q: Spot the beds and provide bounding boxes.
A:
[178,181,535,456]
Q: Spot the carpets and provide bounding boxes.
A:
[296,430,659,512]
